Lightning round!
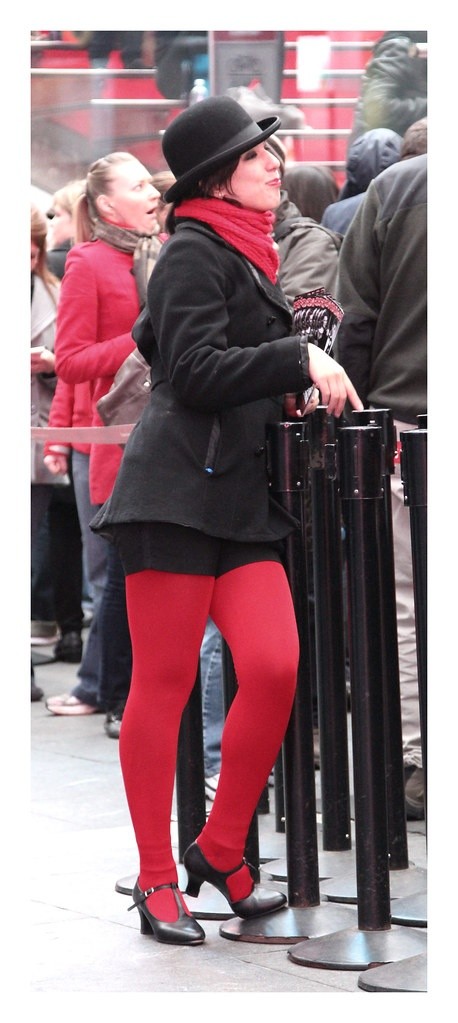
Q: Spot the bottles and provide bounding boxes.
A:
[189,79,208,106]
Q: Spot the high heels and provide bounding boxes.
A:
[183,840,287,920]
[127,876,205,944]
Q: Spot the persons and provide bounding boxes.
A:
[30,30,429,820]
[88,94,363,946]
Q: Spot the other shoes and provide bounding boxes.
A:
[57,627,82,659]
[31,676,43,701]
[105,708,123,737]
[45,692,108,715]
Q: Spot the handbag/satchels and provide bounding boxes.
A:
[97,347,150,450]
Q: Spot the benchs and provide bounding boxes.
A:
[31,40,380,172]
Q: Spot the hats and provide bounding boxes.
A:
[162,95,281,203]
